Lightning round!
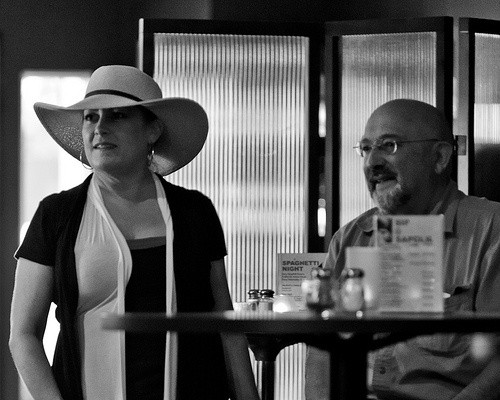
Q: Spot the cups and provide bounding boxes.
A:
[232,302,249,312]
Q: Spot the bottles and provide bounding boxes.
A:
[340,268,365,312]
[310,268,335,312]
[258,290,275,311]
[246,289,259,311]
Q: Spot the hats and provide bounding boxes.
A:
[34,66,208,176]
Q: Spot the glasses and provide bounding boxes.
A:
[353,138,444,158]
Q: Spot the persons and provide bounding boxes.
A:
[8,65,260,400]
[323,99,500,400]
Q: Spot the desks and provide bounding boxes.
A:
[102,311,500,400]
